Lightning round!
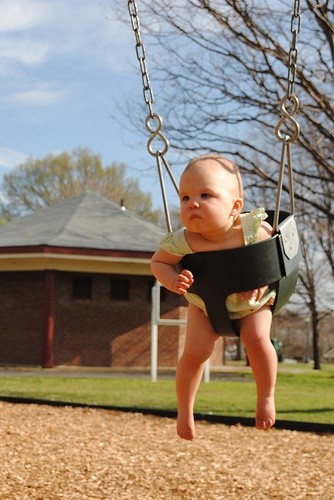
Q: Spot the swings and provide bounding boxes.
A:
[127,0,300,337]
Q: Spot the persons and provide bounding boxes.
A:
[150,152,278,441]
